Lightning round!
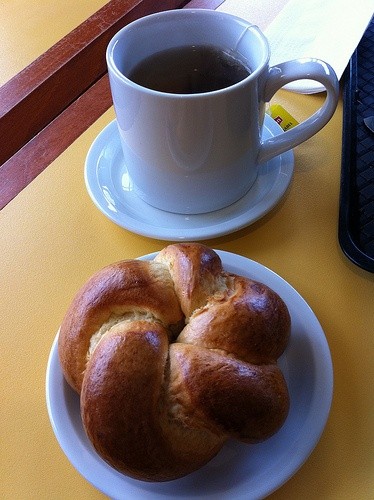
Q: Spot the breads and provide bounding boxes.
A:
[58,242,291,482]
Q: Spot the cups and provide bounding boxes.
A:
[105,10,340,217]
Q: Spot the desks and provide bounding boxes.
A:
[1,1,374,499]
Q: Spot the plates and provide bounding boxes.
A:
[46,243,332,499]
[83,111,296,240]
